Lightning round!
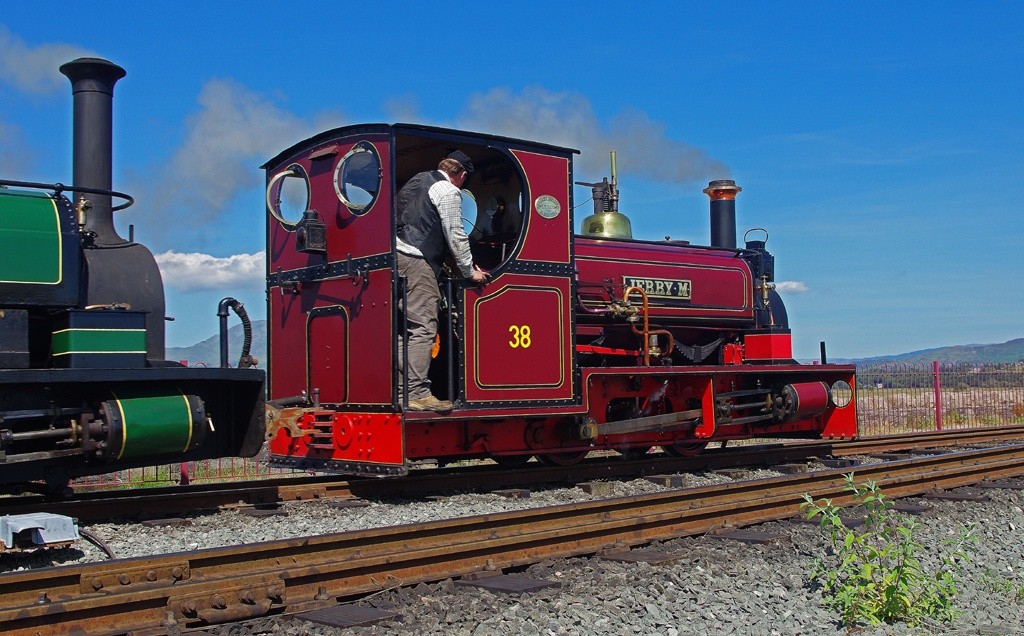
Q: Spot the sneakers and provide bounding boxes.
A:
[408,395,452,411]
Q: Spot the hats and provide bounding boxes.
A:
[448,150,474,174]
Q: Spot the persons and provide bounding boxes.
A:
[395,150,493,412]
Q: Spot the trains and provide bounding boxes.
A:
[0,57,861,486]
